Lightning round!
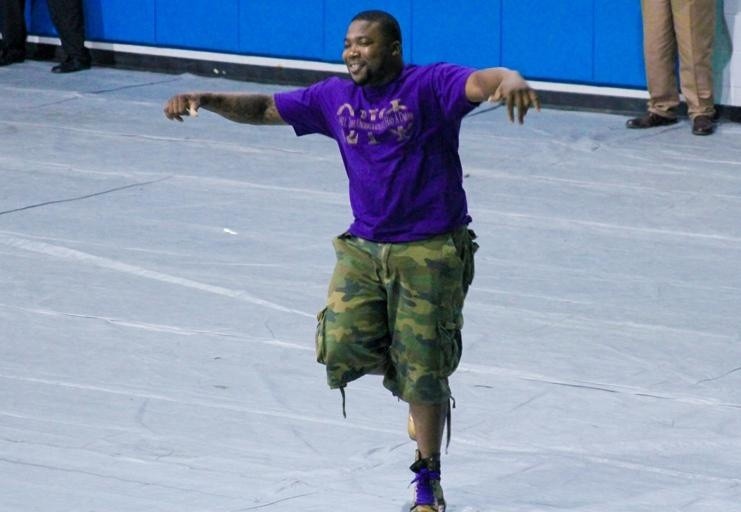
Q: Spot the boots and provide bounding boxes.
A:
[408,450,447,512]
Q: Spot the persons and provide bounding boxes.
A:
[627,0,718,136]
[163,9,547,511]
[0,0,93,74]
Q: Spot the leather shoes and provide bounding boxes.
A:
[691,116,716,135]
[51,56,93,73]
[1,52,28,65]
[625,113,680,128]
